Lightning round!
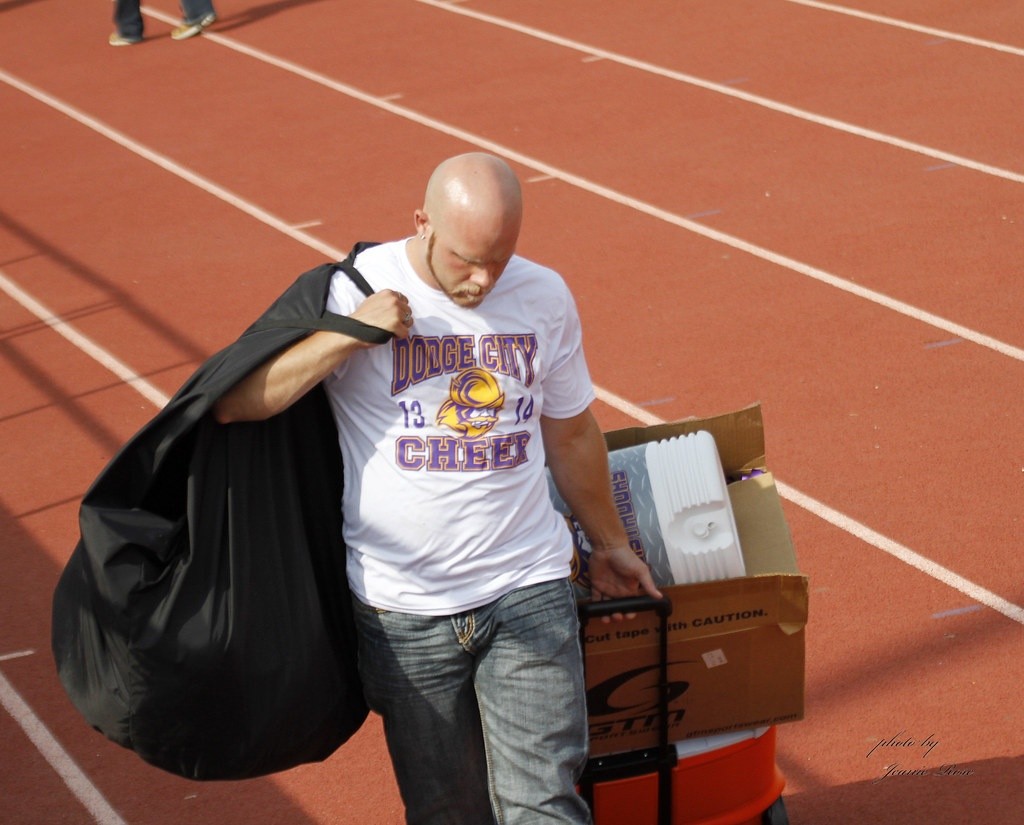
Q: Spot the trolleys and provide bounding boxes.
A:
[533,592,787,825]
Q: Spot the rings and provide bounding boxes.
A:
[396,290,402,299]
[402,312,411,323]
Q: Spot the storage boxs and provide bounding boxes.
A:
[571,399,811,759]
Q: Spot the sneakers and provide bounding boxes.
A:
[170,14,217,39]
[109,32,136,47]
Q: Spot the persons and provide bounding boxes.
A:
[209,151,662,825]
[105,0,220,49]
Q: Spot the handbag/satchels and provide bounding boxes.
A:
[53,236,391,780]
